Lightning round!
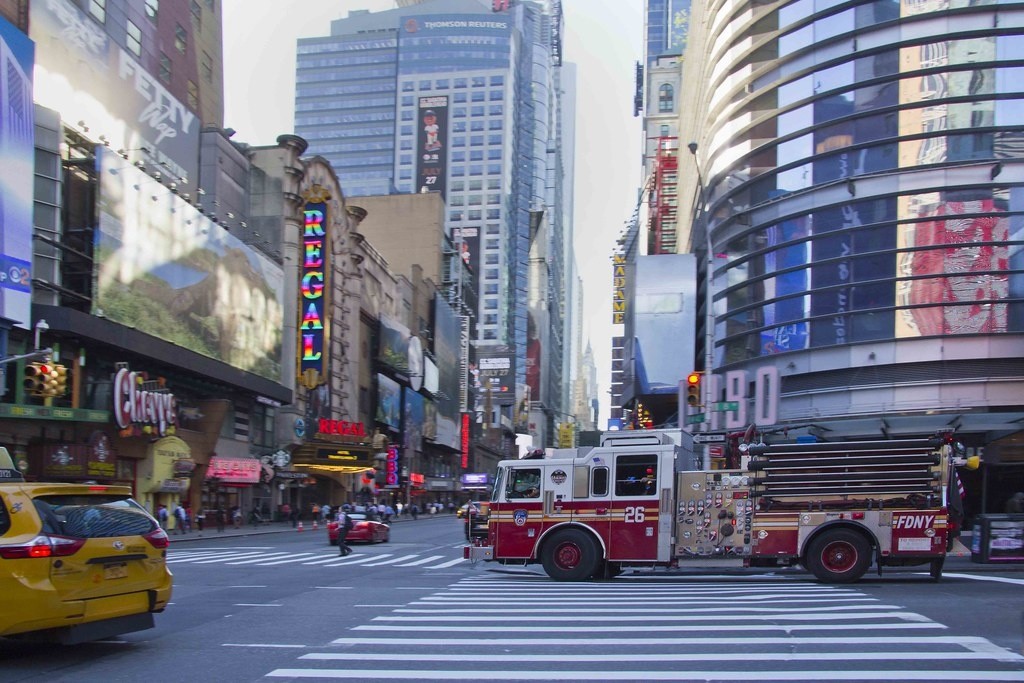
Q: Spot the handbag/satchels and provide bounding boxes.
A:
[237,511,243,519]
[194,517,199,522]
[163,513,167,521]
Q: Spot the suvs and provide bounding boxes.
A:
[0,478,174,646]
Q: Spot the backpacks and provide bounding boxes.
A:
[175,508,180,519]
[341,512,354,532]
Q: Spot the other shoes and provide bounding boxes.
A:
[339,553,347,556]
[346,548,352,555]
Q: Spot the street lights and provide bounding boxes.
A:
[541,406,577,449]
[683,142,713,471]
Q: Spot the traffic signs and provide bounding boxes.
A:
[690,432,726,445]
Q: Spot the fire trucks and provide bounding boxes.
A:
[462,424,956,585]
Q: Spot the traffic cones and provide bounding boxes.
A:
[296,521,304,532]
[325,519,330,527]
[312,519,320,531]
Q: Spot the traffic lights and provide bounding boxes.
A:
[686,374,700,405]
[51,365,71,397]
[38,363,55,394]
[23,363,40,392]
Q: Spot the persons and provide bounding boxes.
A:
[282,503,300,527]
[426,500,444,515]
[253,502,271,527]
[232,506,242,529]
[396,500,419,519]
[343,500,394,519]
[335,506,352,557]
[159,505,169,529]
[173,502,192,535]
[523,485,540,498]
[449,500,454,514]
[313,503,331,520]
[216,504,226,533]
[197,507,206,537]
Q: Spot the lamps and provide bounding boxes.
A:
[78,119,248,232]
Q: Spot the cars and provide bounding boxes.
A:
[328,511,391,543]
[457,504,477,519]
